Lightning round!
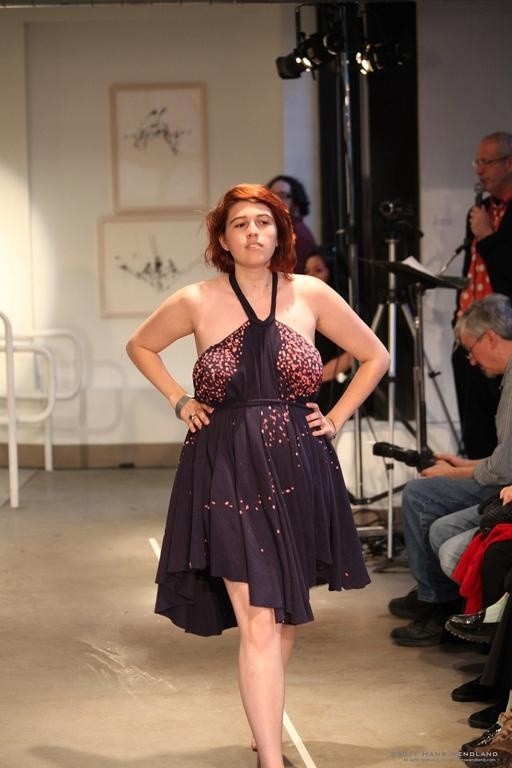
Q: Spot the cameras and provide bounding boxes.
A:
[378,199,414,223]
[372,440,442,471]
[276,28,337,78]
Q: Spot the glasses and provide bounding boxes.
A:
[274,190,296,202]
[464,329,489,359]
[471,155,508,168]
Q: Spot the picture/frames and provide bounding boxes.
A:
[98,215,225,318]
[112,83,209,213]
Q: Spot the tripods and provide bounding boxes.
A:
[330,234,460,573]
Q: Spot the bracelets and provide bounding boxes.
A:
[175,394,194,419]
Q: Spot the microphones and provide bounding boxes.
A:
[473,180,485,211]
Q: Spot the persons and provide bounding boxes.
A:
[466,713,512,768]
[445,569,512,644]
[429,503,481,583]
[443,486,512,654]
[453,594,512,724]
[267,175,316,275]
[462,689,512,752]
[126,182,388,768]
[451,131,512,460]
[304,247,352,416]
[385,295,512,646]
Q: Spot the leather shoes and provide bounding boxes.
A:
[450,676,486,703]
[445,608,500,644]
[460,722,502,753]
[467,705,502,730]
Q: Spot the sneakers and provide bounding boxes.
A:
[474,721,511,755]
[390,619,444,646]
[388,590,434,619]
